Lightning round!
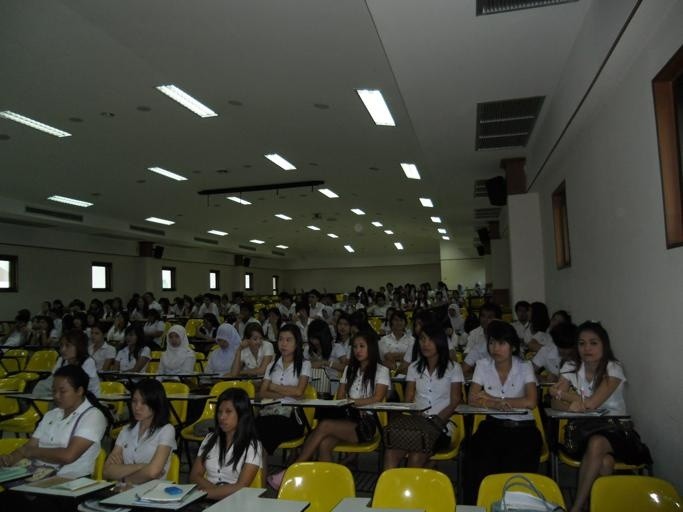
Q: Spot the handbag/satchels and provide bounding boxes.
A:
[311,368,331,395]
[259,398,302,426]
[382,416,444,453]
[313,406,362,422]
[571,419,630,453]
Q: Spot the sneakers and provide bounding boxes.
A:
[266,469,286,491]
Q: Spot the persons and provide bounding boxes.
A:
[1,266,637,512]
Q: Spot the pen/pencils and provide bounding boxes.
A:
[581,389,586,402]
[135,495,141,501]
